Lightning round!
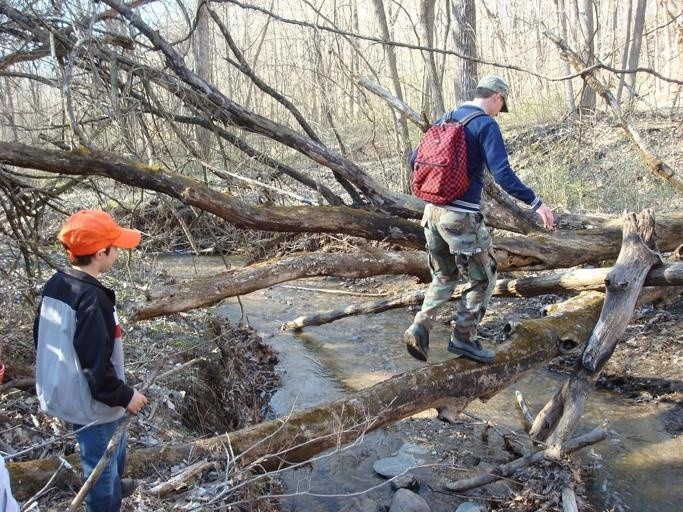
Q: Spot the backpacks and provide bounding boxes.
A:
[410,108,489,205]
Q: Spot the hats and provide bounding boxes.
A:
[476,75,509,112]
[57,210,141,257]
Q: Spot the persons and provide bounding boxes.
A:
[32,208,144,512]
[401,71,555,365]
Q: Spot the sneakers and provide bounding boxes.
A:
[120,476,137,498]
[447,331,496,363]
[404,323,430,361]
